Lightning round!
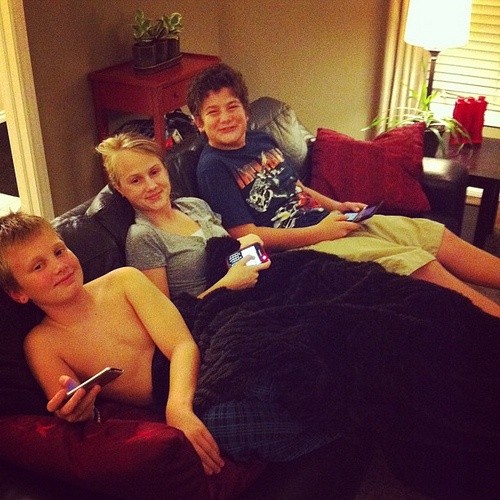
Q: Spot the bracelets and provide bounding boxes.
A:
[93,405,101,422]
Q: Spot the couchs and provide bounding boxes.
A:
[0,97,468,496]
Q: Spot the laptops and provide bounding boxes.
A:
[340,200,384,223]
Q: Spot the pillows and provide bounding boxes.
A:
[306,125,431,214]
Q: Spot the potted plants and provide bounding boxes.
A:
[130,9,182,73]
[358,55,478,156]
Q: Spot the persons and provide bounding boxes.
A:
[95,132,271,300]
[185,63,500,318]
[0,212,226,477]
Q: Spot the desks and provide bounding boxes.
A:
[435,133,500,249]
[85,52,223,157]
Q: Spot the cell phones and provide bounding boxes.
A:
[226,242,268,272]
[62,367,123,404]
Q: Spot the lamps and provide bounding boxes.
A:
[403,0,470,96]
